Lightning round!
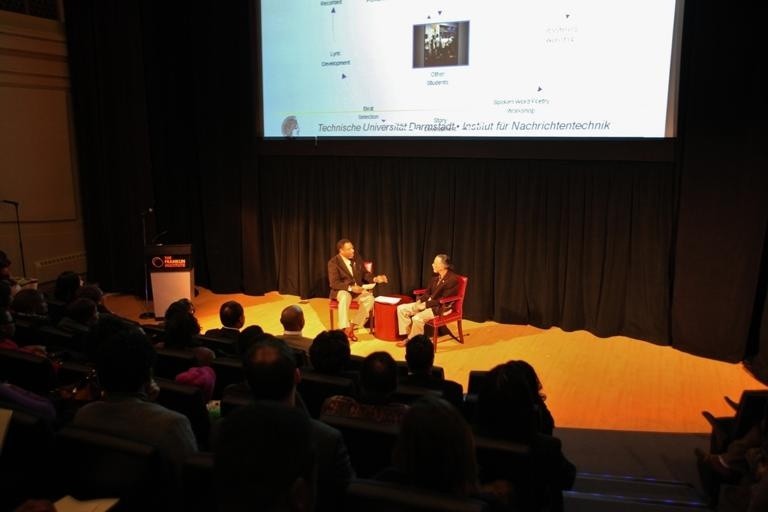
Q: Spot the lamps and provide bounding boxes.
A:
[411,276,468,351]
[329,262,373,330]
[701,389,767,453]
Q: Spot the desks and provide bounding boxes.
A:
[370,294,413,342]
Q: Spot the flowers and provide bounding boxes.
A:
[141,208,153,217]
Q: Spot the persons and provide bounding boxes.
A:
[328,240,388,341]
[396,254,458,346]
[694,417,767,511]
[1,251,573,512]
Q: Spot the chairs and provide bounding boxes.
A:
[0,284,548,512]
[701,389,767,453]
[411,276,468,351]
[329,262,373,330]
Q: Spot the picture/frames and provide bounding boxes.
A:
[370,294,413,342]
